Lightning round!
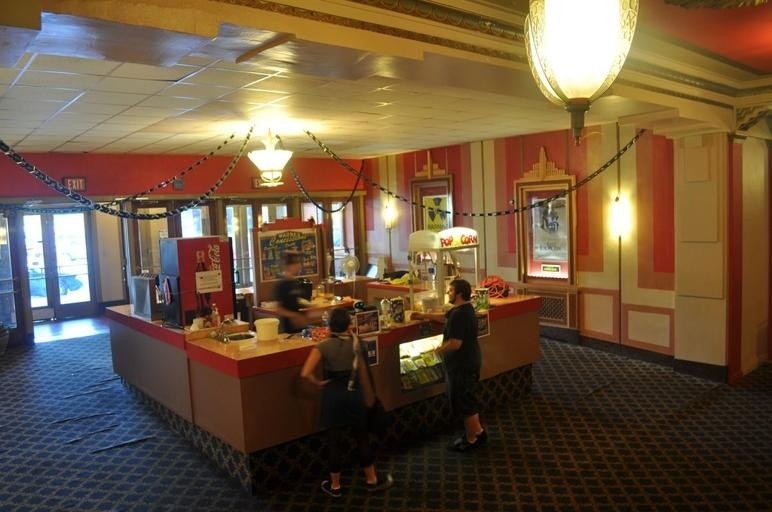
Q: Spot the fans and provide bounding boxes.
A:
[340,253,361,298]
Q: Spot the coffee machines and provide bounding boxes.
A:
[279,277,313,311]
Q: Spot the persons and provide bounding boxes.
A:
[430,279,488,453]
[300,308,395,498]
[273,252,312,334]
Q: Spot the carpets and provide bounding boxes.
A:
[0,331,771,512]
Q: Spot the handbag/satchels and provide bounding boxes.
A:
[365,398,389,434]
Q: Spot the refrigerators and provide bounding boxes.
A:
[158,235,239,330]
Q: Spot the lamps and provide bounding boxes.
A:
[246,133,292,187]
[523,0,642,147]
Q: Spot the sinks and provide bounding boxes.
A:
[225,332,255,341]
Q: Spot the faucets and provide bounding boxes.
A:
[217,319,232,343]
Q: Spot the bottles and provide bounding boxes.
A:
[427,262,436,281]
[318,278,335,298]
[321,311,330,334]
[212,304,220,325]
[195,250,213,318]
[476,289,488,308]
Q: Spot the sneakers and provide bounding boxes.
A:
[475,427,486,444]
[446,432,478,452]
[321,480,342,498]
[364,478,377,491]
